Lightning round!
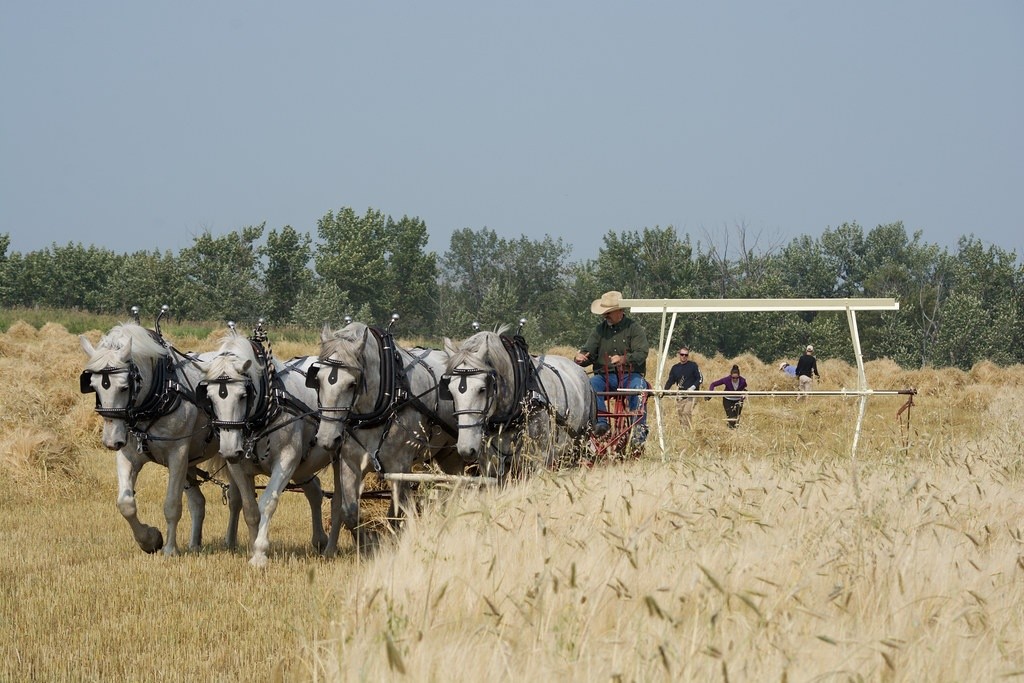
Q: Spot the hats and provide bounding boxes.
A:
[779,362,787,370]
[807,345,813,351]
[591,291,625,314]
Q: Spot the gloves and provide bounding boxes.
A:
[687,385,696,391]
[817,375,820,379]
[740,395,746,400]
[658,392,664,398]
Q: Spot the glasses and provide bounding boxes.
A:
[680,353,689,357]
[732,376,739,379]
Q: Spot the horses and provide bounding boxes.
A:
[75,322,592,567]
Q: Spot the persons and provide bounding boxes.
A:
[779,362,797,378]
[796,345,820,404]
[658,347,703,426]
[574,291,649,457]
[704,365,748,430]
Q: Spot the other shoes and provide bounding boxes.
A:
[594,425,609,435]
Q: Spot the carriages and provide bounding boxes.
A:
[79,298,917,567]
